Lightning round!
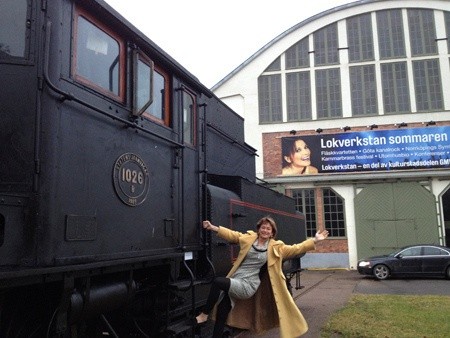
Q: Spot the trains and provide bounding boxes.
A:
[1,0,306,338]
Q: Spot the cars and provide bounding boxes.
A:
[356,244,450,281]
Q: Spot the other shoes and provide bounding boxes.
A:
[186,316,208,328]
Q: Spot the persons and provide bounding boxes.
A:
[281,137,318,175]
[183,216,329,338]
